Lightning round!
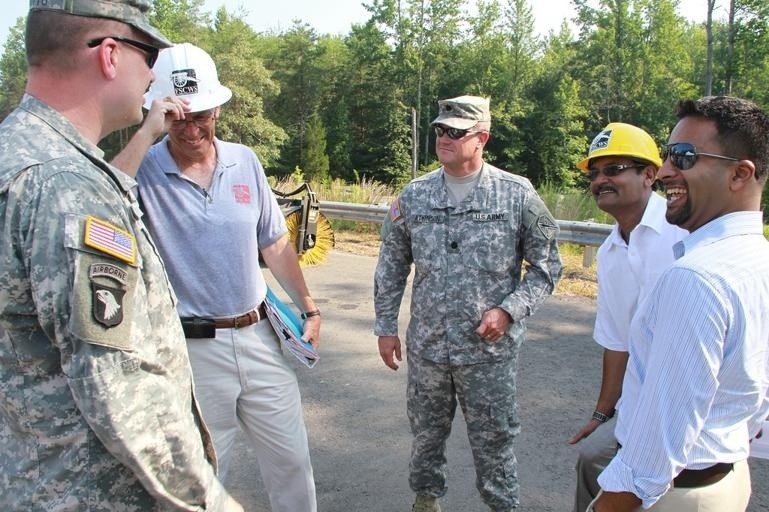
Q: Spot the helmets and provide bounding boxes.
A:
[142,43,232,114]
[576,122,663,180]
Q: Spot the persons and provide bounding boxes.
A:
[1,1,248,511]
[109,42,323,512]
[375,96,562,511]
[569,123,691,446]
[584,96,768,511]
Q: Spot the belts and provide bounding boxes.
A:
[214,308,266,330]
[673,461,733,487]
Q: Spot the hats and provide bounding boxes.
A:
[431,96,489,130]
[30,0,173,48]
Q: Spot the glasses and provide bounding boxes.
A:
[88,37,158,68]
[585,164,646,181]
[171,117,214,130]
[661,143,759,180]
[434,126,481,139]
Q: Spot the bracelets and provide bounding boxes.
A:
[592,410,609,423]
[300,308,322,319]
